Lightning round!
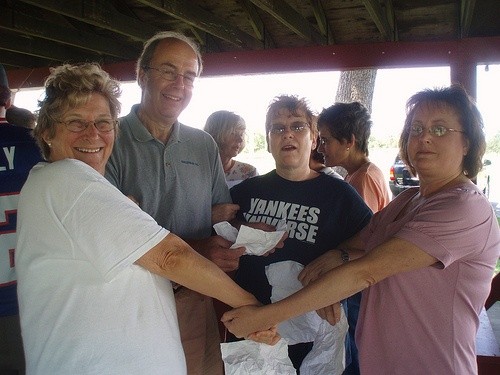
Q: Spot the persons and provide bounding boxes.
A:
[220,82,499,375]
[15,63,281,375]
[204,102,389,214]
[105,33,290,375]
[213,94,373,375]
[1,67,41,375]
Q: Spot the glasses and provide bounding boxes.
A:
[54,118,119,132]
[267,121,311,133]
[146,67,194,87]
[408,125,464,137]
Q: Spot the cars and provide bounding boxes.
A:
[389,151,492,196]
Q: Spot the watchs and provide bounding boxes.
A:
[336,247,352,264]
